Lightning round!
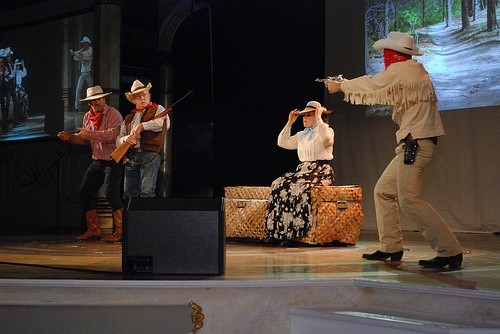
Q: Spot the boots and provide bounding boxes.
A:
[102,208,127,242]
[75,210,103,243]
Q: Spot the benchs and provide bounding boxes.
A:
[212,185,363,247]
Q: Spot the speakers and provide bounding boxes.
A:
[122,197,226,276]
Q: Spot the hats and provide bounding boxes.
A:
[78,85,113,102]
[80,37,90,45]
[125,79,153,103]
[14,59,24,66]
[5,47,14,56]
[296,101,334,116]
[373,31,425,57]
[0,49,10,58]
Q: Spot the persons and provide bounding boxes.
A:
[0,47,29,134]
[256,100,334,247]
[116,79,170,197]
[69,36,93,112]
[57,85,123,241]
[324,32,464,269]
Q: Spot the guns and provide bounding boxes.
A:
[315,74,343,89]
[109,90,194,163]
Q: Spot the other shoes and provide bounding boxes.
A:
[362,249,404,262]
[417,253,463,270]
[264,237,277,243]
[280,239,300,248]
